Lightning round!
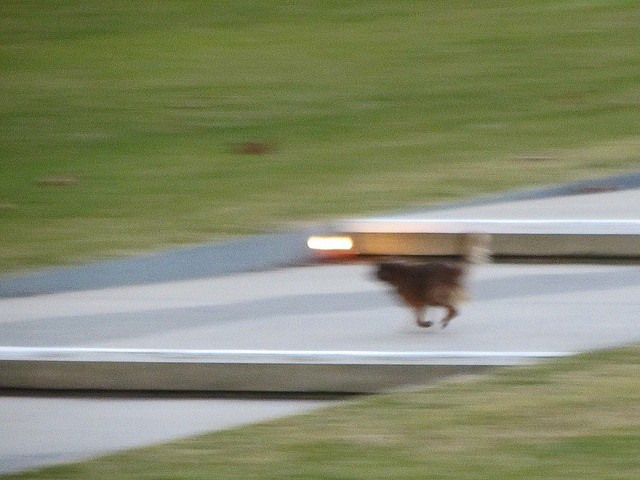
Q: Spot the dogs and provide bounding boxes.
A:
[375,228,488,328]
